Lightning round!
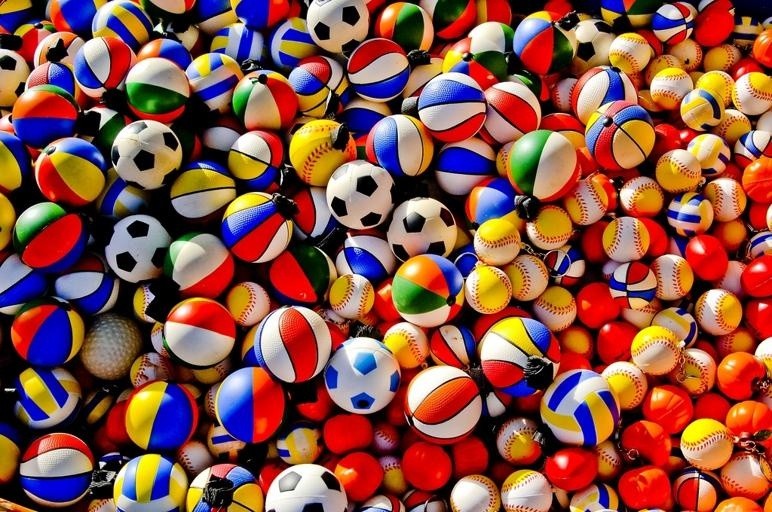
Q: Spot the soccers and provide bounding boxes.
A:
[0,0,771,512]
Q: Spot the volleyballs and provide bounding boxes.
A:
[542,372,619,446]
